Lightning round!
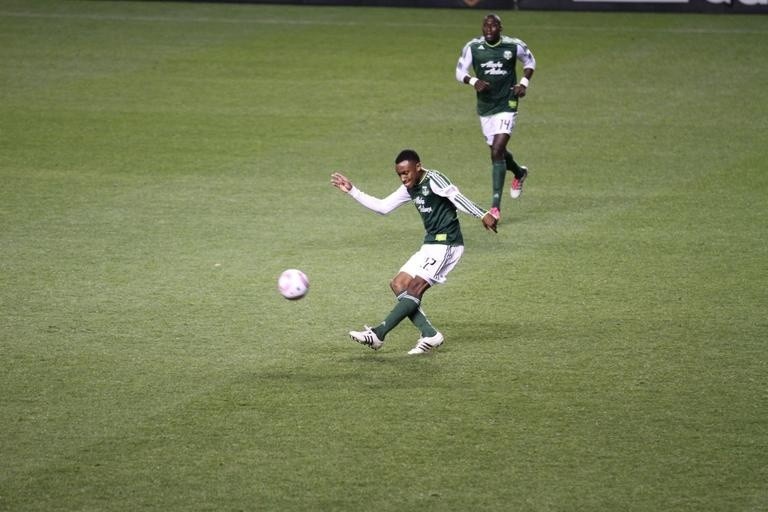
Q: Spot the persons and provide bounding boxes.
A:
[329,149,498,355]
[453,14,538,222]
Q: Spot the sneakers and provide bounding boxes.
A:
[510,165,528,200]
[406,331,445,356]
[349,326,384,351]
[488,207,501,222]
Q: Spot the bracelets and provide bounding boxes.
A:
[469,77,479,86]
[520,76,529,88]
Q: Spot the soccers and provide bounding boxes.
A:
[278,268,310,299]
